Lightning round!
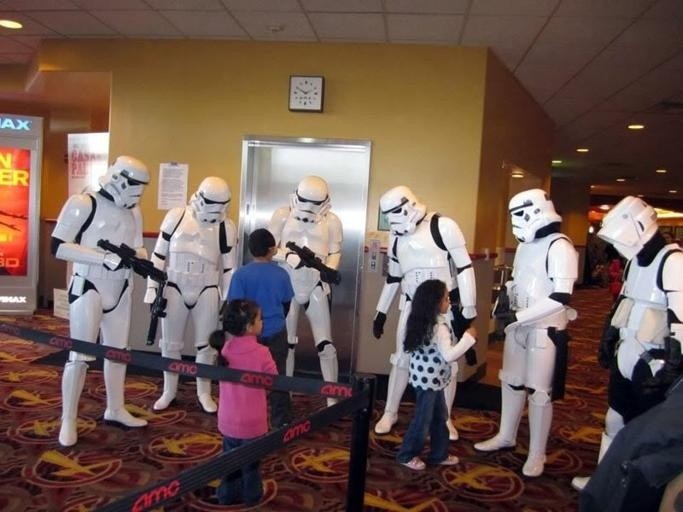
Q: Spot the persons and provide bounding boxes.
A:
[571,195,683,494]
[267,175,344,407]
[472,188,579,479]
[143,176,238,413]
[208,299,280,505]
[395,279,479,471]
[49,155,151,447]
[372,185,476,440]
[595,231,674,304]
[226,228,295,426]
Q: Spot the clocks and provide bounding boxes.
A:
[287,74,323,115]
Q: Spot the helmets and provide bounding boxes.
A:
[190,176,231,225]
[508,189,562,243]
[596,196,658,261]
[99,156,148,208]
[379,185,425,235]
[290,177,330,223]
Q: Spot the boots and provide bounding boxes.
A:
[521,390,552,477]
[571,432,612,490]
[286,347,295,397]
[443,363,458,441]
[195,345,219,414]
[473,381,526,452]
[58,362,89,447]
[103,358,147,428]
[374,353,410,434]
[153,350,181,411]
[317,344,338,407]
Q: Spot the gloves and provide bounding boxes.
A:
[655,337,680,391]
[495,312,515,326]
[372,311,385,339]
[598,326,619,369]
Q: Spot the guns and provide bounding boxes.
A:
[96,239,167,286]
[146,283,167,346]
[491,284,512,339]
[450,291,476,366]
[285,240,342,285]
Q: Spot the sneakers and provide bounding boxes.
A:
[438,455,458,466]
[400,457,426,471]
